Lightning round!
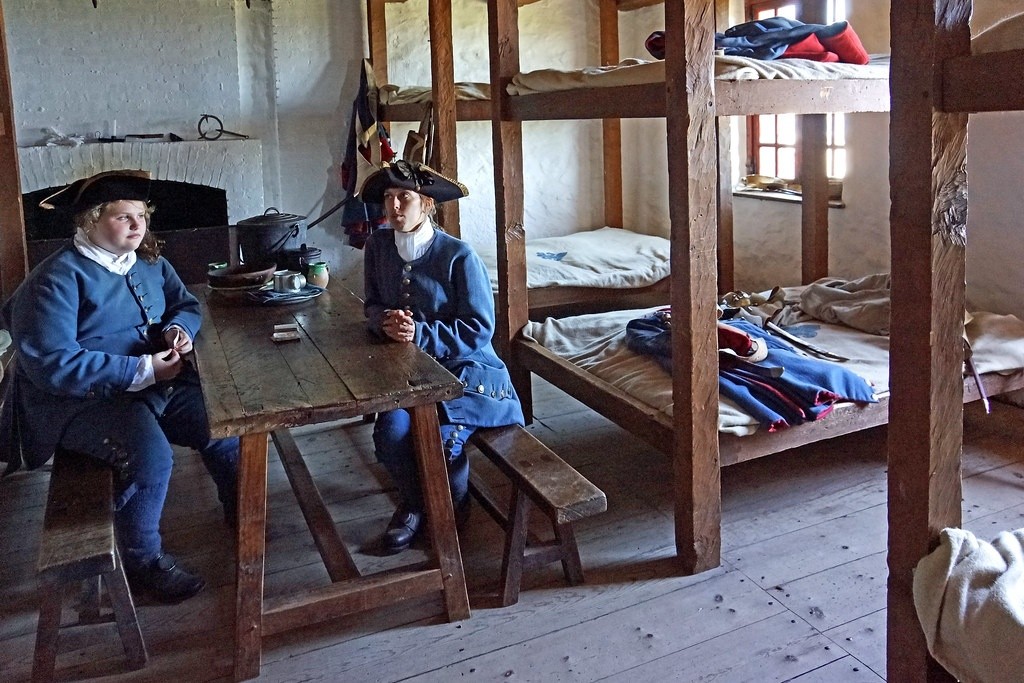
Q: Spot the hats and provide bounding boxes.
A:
[38,169,155,212]
[358,159,469,204]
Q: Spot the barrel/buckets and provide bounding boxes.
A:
[237,208,308,270]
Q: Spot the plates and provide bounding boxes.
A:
[260,285,325,304]
[207,281,273,297]
[207,264,277,287]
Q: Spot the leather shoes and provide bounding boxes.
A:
[123,556,208,602]
[381,504,428,554]
[457,501,476,530]
[224,506,242,528]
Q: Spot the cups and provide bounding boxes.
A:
[308,262,330,289]
[273,271,301,293]
[208,262,227,271]
[286,243,322,282]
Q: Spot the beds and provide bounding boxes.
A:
[366,2,1024,683]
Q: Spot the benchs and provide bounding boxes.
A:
[29,450,147,683]
[466,422,609,608]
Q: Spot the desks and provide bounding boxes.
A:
[180,272,472,682]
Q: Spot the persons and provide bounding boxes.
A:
[359,163,527,553]
[0,169,240,606]
